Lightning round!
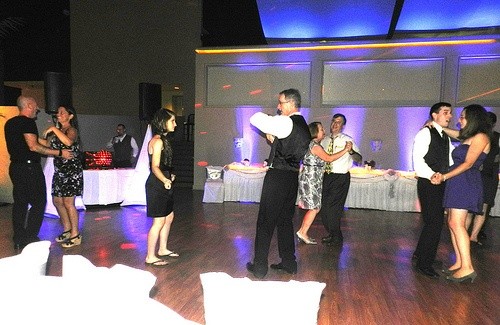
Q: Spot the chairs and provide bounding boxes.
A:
[202,166,224,203]
[62,255,156,297]
[199,272,326,325]
[183,114,194,140]
[0,239,52,276]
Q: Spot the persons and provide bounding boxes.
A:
[294,122,350,245]
[425,104,492,284]
[105,124,139,167]
[145,107,177,266]
[465,111,500,245]
[411,102,456,278]
[4,94,74,249]
[37,102,84,247]
[247,88,312,279]
[319,113,362,246]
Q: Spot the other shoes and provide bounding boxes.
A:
[15,243,21,249]
[479,231,486,238]
[61,233,82,247]
[296,232,317,245]
[55,229,72,242]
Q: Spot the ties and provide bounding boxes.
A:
[325,135,338,176]
[441,129,447,141]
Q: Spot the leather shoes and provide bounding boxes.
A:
[322,237,332,241]
[271,263,297,274]
[327,240,343,246]
[246,262,264,278]
[417,264,438,279]
[412,254,442,265]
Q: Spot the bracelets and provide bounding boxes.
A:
[58,149,62,156]
[443,175,446,181]
[349,150,355,155]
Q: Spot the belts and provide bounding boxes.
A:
[24,160,40,164]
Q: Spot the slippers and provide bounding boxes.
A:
[145,259,168,267]
[157,251,179,258]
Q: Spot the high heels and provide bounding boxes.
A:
[446,271,477,284]
[441,268,460,273]
[471,240,483,248]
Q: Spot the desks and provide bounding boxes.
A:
[0,275,201,325]
[82,167,135,206]
[223,164,423,213]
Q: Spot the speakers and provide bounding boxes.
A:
[138,82,162,120]
[43,71,72,113]
